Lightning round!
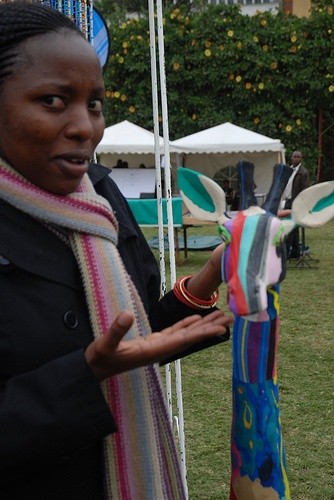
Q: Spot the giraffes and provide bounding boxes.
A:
[177,160,334,500]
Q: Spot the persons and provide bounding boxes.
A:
[287,151,309,256]
[0,1,234,499]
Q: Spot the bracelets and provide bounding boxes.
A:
[174,275,220,312]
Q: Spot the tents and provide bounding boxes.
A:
[168,122,287,210]
[88,119,164,169]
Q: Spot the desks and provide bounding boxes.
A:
[176,218,319,268]
